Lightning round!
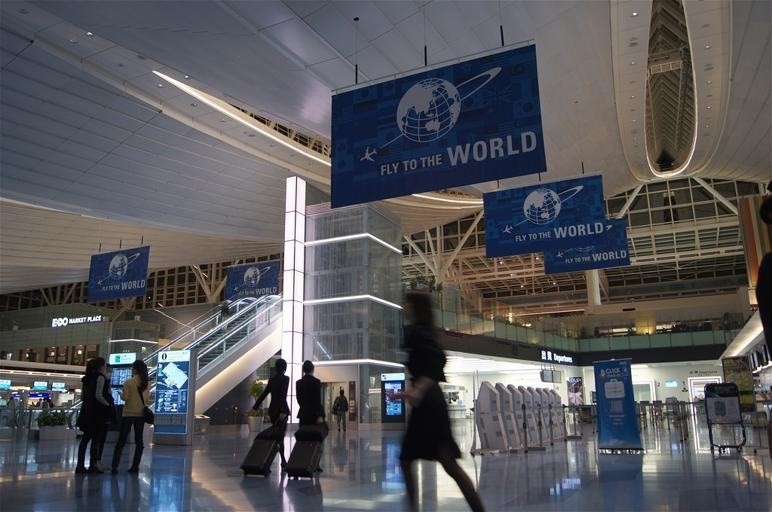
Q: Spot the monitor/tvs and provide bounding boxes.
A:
[34,381,47,391]
[0,380,12,391]
[52,381,65,392]
[541,370,562,382]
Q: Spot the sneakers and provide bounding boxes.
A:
[70,462,106,475]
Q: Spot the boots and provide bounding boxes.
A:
[127,446,145,476]
[110,446,125,476]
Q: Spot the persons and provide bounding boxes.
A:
[75,357,149,473]
[220,301,228,331]
[5,396,54,408]
[386,293,486,512]
[296,360,325,473]
[333,390,349,432]
[245,359,290,472]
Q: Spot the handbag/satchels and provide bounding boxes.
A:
[141,403,156,426]
[255,420,286,439]
[294,422,330,441]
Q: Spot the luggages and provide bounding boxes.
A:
[240,439,281,481]
[282,440,328,483]
